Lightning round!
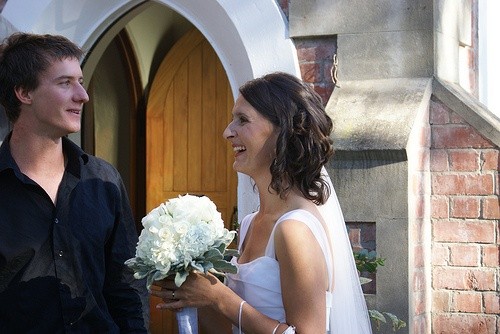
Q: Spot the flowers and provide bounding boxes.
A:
[124,193,240,286]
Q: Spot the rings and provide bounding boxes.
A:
[172,291,175,298]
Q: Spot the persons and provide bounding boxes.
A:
[148,73,372,334]
[0,31,152,334]
